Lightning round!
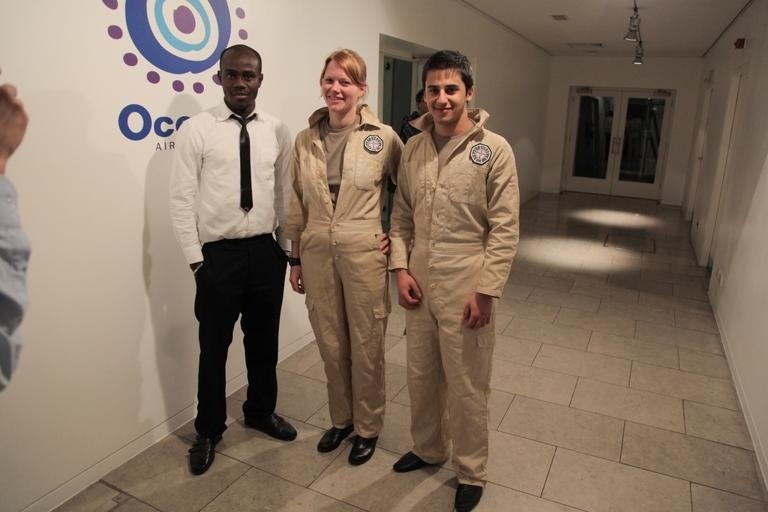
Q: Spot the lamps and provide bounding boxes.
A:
[621,2,644,65]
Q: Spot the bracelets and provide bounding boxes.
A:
[288,258,301,266]
[193,262,204,275]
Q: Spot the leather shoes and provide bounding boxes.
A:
[187,430,224,476]
[392,449,447,473]
[347,433,379,466]
[316,421,355,453]
[243,410,298,442]
[454,482,483,512]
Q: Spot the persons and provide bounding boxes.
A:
[170,45,297,475]
[289,49,406,466]
[400,90,428,144]
[0,84,31,390]
[387,51,521,512]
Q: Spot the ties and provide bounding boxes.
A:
[230,113,259,214]
